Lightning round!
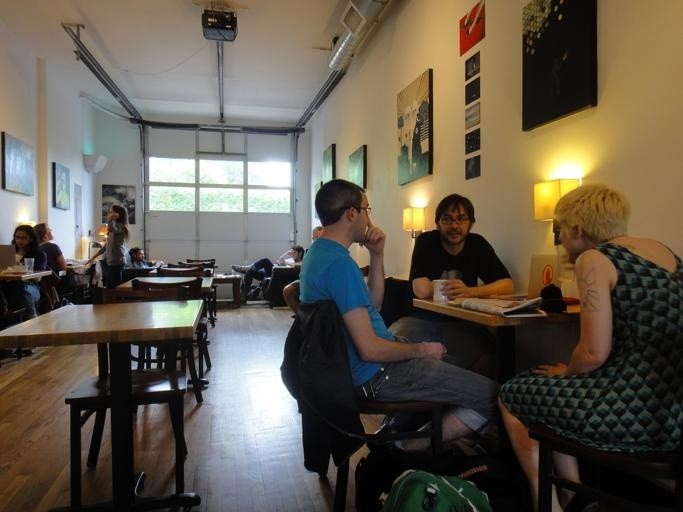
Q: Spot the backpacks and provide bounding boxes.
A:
[379,469,492,512]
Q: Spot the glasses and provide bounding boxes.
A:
[439,215,469,224]
[338,207,371,219]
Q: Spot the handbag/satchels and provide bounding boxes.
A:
[247,281,264,300]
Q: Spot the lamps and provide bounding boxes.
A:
[402,207,426,238]
[534,180,581,222]
[97,224,110,239]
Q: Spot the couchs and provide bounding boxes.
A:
[261,266,301,307]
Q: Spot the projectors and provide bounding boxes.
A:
[201,9,238,42]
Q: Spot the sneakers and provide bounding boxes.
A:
[367,440,404,470]
[240,297,247,304]
[232,265,246,274]
[564,493,598,512]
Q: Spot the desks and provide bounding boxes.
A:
[212,275,242,308]
[413,298,583,328]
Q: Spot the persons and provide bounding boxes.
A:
[0,225,47,319]
[232,245,305,305]
[35,223,66,279]
[300,179,506,455]
[497,184,683,511]
[283,226,323,318]
[128,246,164,276]
[85,204,130,290]
[387,194,516,379]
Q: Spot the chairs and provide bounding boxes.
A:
[527,426,683,512]
[292,298,442,511]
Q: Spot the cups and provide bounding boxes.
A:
[24,258,34,273]
[433,279,448,302]
